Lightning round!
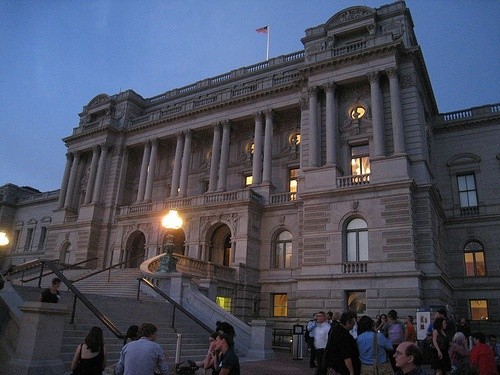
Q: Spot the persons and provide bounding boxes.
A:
[38,278,62,303]
[307,309,500,375]
[70,321,240,375]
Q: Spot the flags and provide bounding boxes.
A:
[257,25,268,35]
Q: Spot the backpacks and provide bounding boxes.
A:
[388,321,403,343]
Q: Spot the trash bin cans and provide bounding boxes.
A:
[292,325,304,360]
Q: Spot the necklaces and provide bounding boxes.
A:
[51,289,56,294]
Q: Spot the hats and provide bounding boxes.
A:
[216,321,235,336]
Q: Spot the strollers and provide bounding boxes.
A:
[175,360,199,375]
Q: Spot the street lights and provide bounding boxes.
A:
[158,210,183,272]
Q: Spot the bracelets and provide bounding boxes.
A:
[208,350,213,354]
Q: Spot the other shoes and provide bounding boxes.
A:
[310,363,318,368]
